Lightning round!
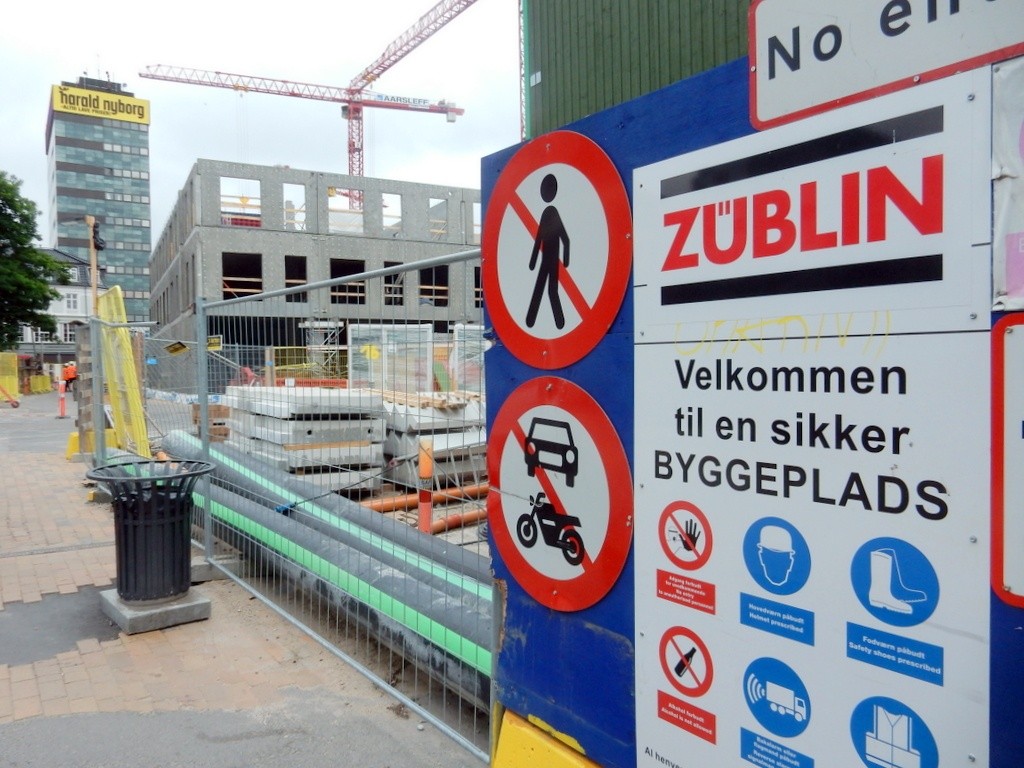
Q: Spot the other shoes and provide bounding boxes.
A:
[66,390,72,392]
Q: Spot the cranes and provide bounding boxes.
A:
[139,62,465,211]
[348,0,478,96]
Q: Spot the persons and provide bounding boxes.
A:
[61,361,77,392]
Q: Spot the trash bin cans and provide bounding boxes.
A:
[87,458,217,602]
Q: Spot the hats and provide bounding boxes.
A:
[64,363,68,367]
[69,361,74,366]
